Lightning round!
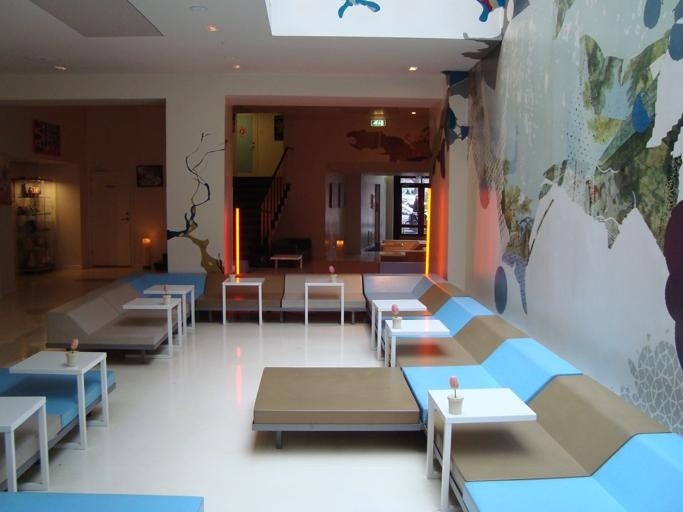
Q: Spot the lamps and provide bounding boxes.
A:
[368,109,386,129]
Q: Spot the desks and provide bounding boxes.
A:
[369,299,427,360]
[303,279,345,326]
[424,389,538,510]
[221,277,265,326]
[9,350,109,451]
[0,396,50,491]
[383,319,452,367]
[270,254,303,270]
[124,297,183,359]
[144,284,195,334]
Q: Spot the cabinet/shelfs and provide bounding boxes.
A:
[17,177,56,275]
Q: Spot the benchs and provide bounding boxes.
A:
[188,271,367,325]
[0,366,117,491]
[362,271,681,510]
[252,366,423,449]
[46,271,207,357]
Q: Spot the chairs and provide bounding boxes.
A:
[272,236,312,263]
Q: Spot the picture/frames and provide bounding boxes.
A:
[135,165,162,187]
